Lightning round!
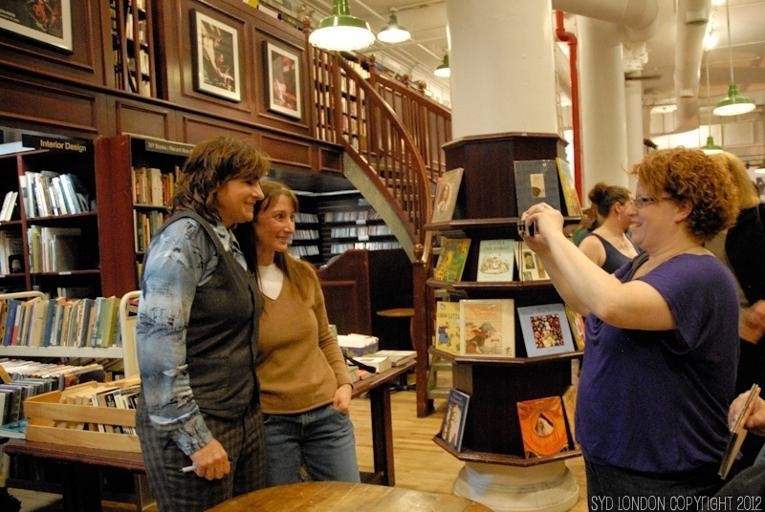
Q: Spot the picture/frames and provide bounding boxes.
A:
[260,39,304,121]
[189,8,242,105]
[512,303,576,358]
[0,1,72,57]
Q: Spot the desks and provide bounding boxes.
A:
[330,351,419,486]
[3,419,153,501]
[204,480,496,511]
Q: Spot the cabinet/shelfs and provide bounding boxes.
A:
[132,161,194,280]
[312,47,368,150]
[418,208,594,366]
[107,0,158,100]
[21,146,136,298]
[0,286,125,361]
[284,194,328,258]
[323,198,414,256]
[1,157,29,296]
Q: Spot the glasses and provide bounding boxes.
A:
[629,192,680,209]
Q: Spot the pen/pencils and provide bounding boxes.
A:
[178,456,233,473]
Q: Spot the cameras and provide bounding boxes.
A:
[516,220,535,237]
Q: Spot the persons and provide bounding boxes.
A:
[233,180,360,487]
[136,135,269,512]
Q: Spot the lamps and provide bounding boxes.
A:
[711,0,757,115]
[379,10,413,45]
[433,47,451,78]
[306,0,378,54]
[701,61,725,159]
